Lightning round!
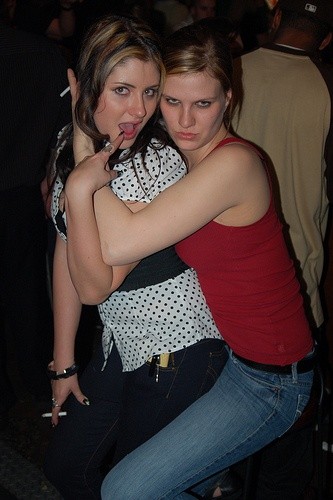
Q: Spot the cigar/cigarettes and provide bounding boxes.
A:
[42,412,67,417]
[60,85,70,97]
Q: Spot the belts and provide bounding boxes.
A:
[232,348,318,375]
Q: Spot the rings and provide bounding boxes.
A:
[102,142,112,152]
[52,404,63,407]
[51,398,57,404]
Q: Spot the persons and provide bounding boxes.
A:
[47,17,230,500]
[227,0,333,327]
[66,39,315,500]
[162,0,218,36]
[39,0,84,114]
[0,0,57,431]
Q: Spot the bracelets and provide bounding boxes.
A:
[48,360,78,380]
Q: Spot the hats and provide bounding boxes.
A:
[271,0,333,31]
